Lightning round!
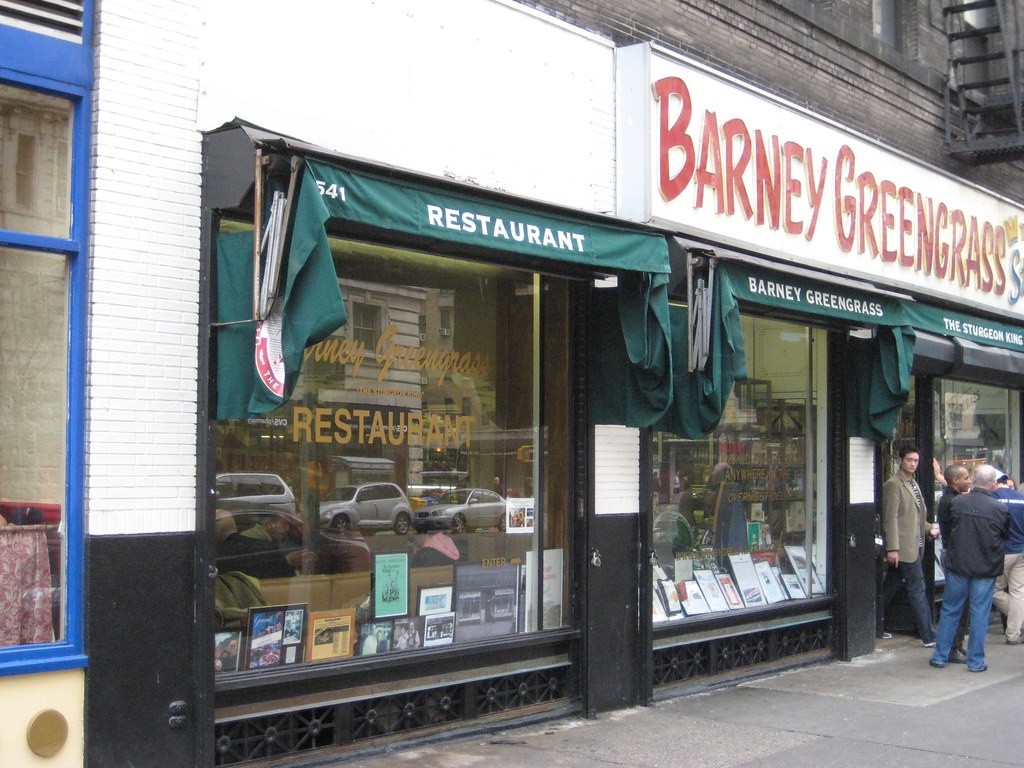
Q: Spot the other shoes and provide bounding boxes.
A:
[923,641,936,647]
[968,664,987,672]
[1007,640,1017,644]
[949,649,967,663]
[880,632,892,639]
[930,659,944,668]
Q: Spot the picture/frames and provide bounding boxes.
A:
[369,548,411,621]
[305,608,356,662]
[356,617,396,656]
[214,627,245,673]
[241,603,308,671]
[416,583,455,617]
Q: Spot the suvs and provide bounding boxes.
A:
[320,483,412,533]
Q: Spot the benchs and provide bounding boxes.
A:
[250,562,455,614]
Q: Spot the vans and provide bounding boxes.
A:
[214,472,295,519]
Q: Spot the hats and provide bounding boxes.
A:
[995,469,1009,482]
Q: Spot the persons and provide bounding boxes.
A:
[881,447,937,646]
[285,612,301,638]
[933,458,1024,644]
[490,596,512,614]
[215,640,239,672]
[251,616,281,658]
[653,463,749,570]
[316,629,332,644]
[382,581,399,601]
[463,597,479,618]
[495,477,503,497]
[394,621,420,650]
[426,623,452,638]
[762,466,787,546]
[407,519,461,568]
[426,596,442,610]
[938,464,971,662]
[930,465,1010,671]
[214,509,311,578]
[360,623,387,654]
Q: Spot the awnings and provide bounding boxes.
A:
[667,238,915,441]
[205,128,674,429]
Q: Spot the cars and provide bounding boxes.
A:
[214,508,370,581]
[413,488,509,531]
